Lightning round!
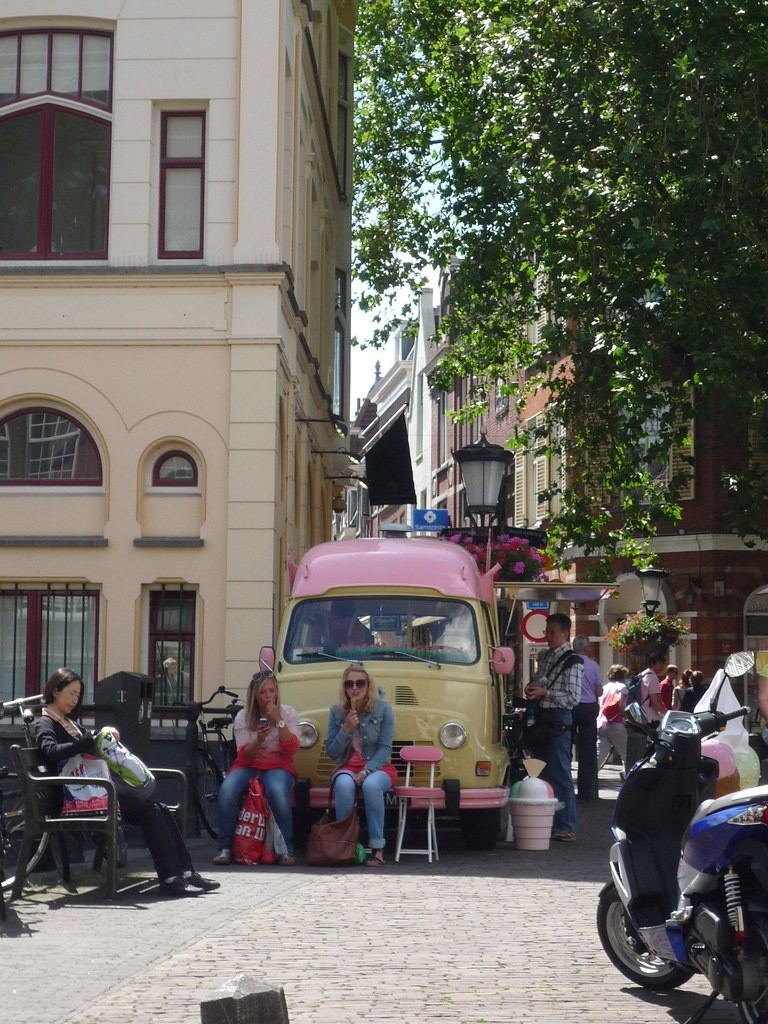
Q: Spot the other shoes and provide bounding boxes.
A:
[212,849,232,865]
[554,830,578,842]
[277,855,297,867]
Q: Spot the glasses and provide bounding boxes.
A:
[671,671,679,675]
[249,669,273,681]
[344,678,366,690]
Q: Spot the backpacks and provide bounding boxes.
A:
[621,670,653,708]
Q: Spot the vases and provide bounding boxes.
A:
[628,636,650,656]
[660,631,677,647]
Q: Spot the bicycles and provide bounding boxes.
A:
[172,684,245,841]
[0,694,52,896]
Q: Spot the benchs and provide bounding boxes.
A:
[11,743,189,910]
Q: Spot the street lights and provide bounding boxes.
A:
[636,563,671,672]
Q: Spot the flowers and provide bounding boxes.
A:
[603,613,657,654]
[661,613,691,634]
[451,533,557,581]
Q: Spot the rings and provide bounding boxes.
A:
[355,718,357,721]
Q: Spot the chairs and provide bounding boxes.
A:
[389,747,446,864]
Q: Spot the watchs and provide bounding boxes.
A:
[276,720,286,728]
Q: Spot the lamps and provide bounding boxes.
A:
[292,419,348,438]
[685,572,704,607]
[322,476,373,490]
[309,449,366,464]
[570,602,585,612]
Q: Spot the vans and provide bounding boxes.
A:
[256,517,517,849]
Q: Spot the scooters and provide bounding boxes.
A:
[595,649,768,1024]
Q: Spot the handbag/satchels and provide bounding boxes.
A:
[90,728,156,801]
[260,809,278,865]
[603,694,626,724]
[231,774,268,866]
[269,817,291,859]
[58,752,125,819]
[304,803,364,865]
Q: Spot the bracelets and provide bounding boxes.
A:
[366,769,370,776]
[544,691,550,701]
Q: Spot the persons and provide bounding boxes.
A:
[671,669,694,710]
[213,670,301,866]
[34,668,220,897]
[681,671,709,713]
[596,664,630,772]
[524,613,585,841]
[637,653,667,730]
[326,664,397,867]
[572,635,603,802]
[659,664,679,710]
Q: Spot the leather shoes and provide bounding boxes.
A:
[157,877,206,897]
[183,872,221,892]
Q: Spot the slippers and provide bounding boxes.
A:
[363,847,388,868]
